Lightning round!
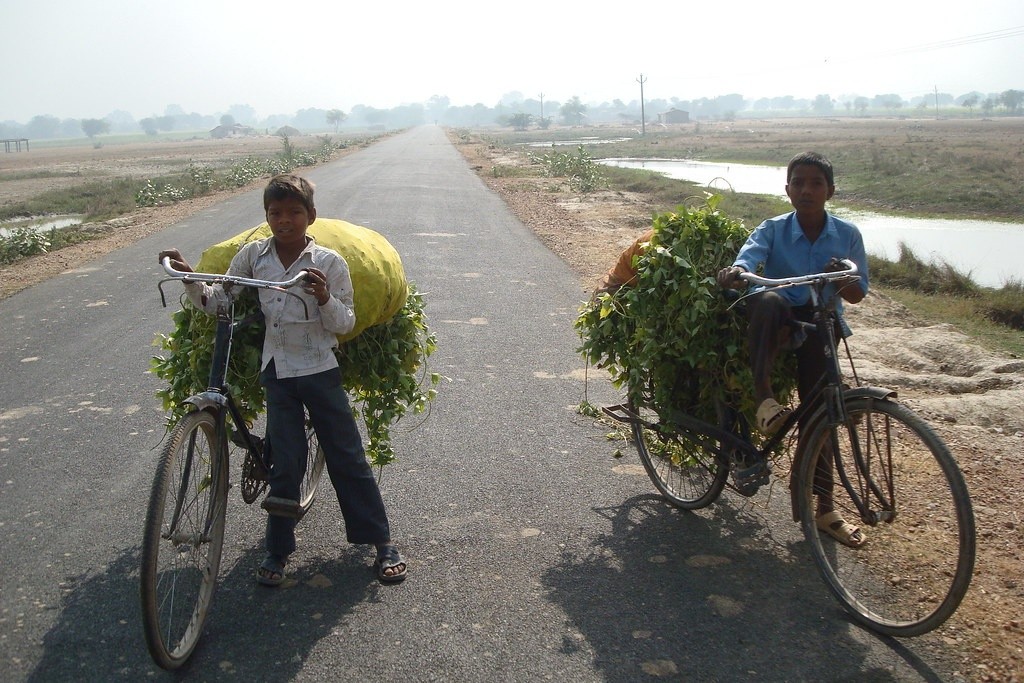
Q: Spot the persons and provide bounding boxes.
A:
[159,176,408,586]
[717,153,869,546]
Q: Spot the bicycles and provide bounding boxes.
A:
[592,255,981,639]
[135,256,327,670]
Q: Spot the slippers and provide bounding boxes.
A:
[374,544,407,580]
[756,398,794,439]
[256,552,286,584]
[815,510,867,547]
